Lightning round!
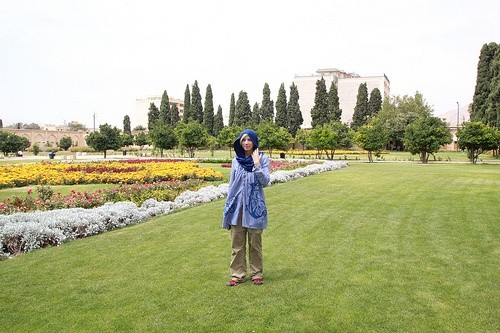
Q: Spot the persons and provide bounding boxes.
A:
[222,129,270,286]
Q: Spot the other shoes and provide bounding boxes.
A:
[226,276,247,286]
[252,278,263,284]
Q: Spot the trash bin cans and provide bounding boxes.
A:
[123,151,126,155]
[49,154,55,159]
[280,153,285,158]
